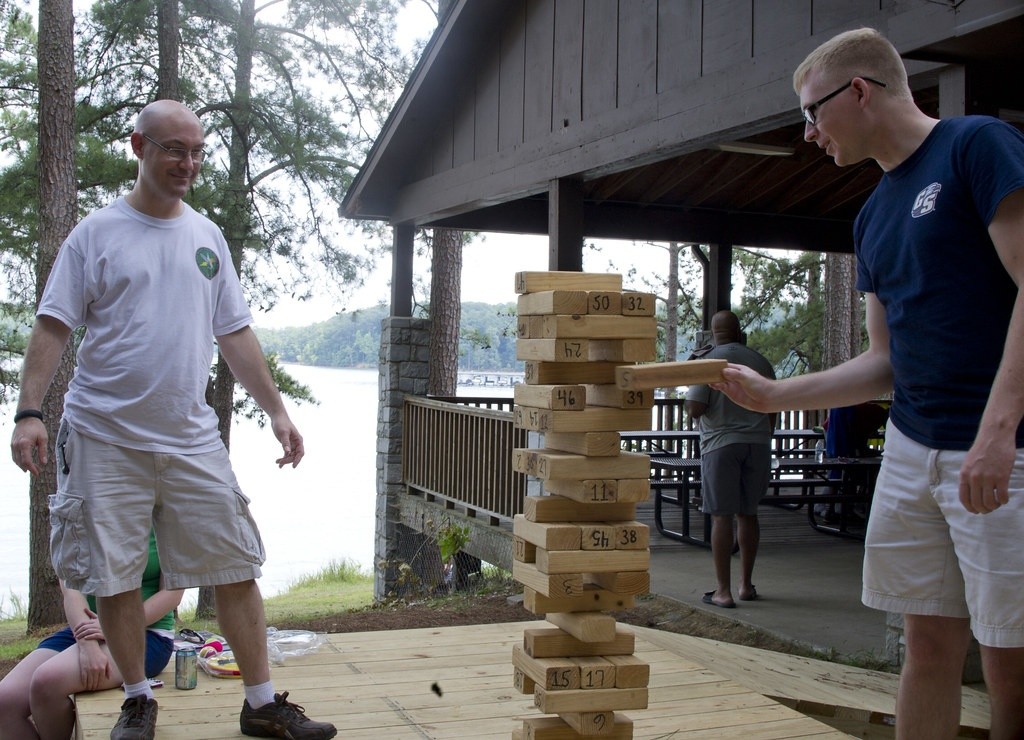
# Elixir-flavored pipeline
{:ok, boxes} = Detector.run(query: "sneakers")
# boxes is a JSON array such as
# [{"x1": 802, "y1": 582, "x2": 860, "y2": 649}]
[
  {"x1": 109, "y1": 693, "x2": 159, "y2": 740},
  {"x1": 239, "y1": 691, "x2": 337, "y2": 740}
]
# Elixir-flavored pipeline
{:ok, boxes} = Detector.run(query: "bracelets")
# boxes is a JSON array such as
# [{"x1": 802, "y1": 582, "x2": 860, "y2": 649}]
[{"x1": 14, "y1": 409, "x2": 44, "y2": 423}]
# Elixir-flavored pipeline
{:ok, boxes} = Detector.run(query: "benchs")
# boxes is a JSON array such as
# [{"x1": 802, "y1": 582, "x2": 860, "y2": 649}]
[
  {"x1": 771, "y1": 449, "x2": 826, "y2": 458},
  {"x1": 692, "y1": 493, "x2": 873, "y2": 554},
  {"x1": 650, "y1": 479, "x2": 842, "y2": 530}
]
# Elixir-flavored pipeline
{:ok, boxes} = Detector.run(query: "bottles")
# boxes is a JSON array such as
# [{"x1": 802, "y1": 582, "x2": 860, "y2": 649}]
[{"x1": 815, "y1": 440, "x2": 823, "y2": 463}]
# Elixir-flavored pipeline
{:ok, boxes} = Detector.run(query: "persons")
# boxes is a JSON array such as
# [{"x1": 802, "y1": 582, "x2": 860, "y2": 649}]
[
  {"x1": 684, "y1": 28, "x2": 1024, "y2": 740},
  {"x1": 0, "y1": 100, "x2": 337, "y2": 740}
]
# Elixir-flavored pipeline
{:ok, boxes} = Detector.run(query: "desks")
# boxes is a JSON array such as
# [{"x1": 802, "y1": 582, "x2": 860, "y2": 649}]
[
  {"x1": 618, "y1": 431, "x2": 825, "y2": 504},
  {"x1": 649, "y1": 456, "x2": 881, "y2": 554}
]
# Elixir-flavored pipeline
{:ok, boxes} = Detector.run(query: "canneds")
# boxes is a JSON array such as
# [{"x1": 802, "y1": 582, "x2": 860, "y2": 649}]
[{"x1": 175, "y1": 648, "x2": 197, "y2": 690}]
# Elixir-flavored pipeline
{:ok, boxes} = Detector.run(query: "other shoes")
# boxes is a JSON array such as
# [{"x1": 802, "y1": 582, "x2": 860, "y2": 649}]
[
  {"x1": 701, "y1": 589, "x2": 736, "y2": 609},
  {"x1": 738, "y1": 584, "x2": 757, "y2": 601}
]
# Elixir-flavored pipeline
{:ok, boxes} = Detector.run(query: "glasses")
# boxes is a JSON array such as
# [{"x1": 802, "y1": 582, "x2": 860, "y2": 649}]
[
  {"x1": 801, "y1": 77, "x2": 886, "y2": 126},
  {"x1": 143, "y1": 134, "x2": 215, "y2": 159}
]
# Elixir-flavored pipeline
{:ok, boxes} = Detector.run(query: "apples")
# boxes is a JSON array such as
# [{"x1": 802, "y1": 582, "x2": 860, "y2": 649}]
[{"x1": 200, "y1": 638, "x2": 219, "y2": 657}]
